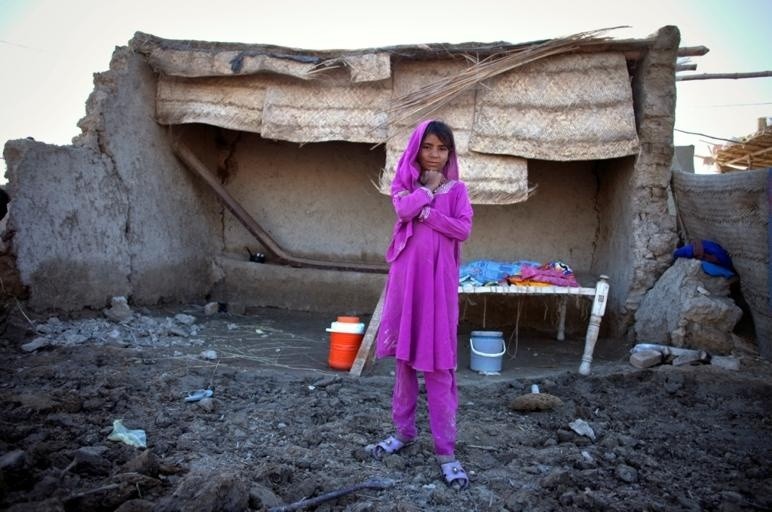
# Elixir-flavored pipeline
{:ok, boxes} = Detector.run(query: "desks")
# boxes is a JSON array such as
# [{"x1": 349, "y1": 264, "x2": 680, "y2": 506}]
[{"x1": 456, "y1": 273, "x2": 610, "y2": 374}]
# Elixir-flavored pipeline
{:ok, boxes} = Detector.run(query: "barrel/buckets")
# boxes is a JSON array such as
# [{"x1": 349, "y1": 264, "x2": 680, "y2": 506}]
[
  {"x1": 325, "y1": 314, "x2": 365, "y2": 369},
  {"x1": 469, "y1": 331, "x2": 507, "y2": 373}
]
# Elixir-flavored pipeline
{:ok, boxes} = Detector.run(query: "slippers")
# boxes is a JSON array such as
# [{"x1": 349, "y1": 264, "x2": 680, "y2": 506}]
[
  {"x1": 373, "y1": 434, "x2": 415, "y2": 462},
  {"x1": 438, "y1": 459, "x2": 469, "y2": 492}
]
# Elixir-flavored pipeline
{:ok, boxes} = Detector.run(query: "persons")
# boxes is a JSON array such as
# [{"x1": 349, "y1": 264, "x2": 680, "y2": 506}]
[{"x1": 370, "y1": 120, "x2": 474, "y2": 494}]
